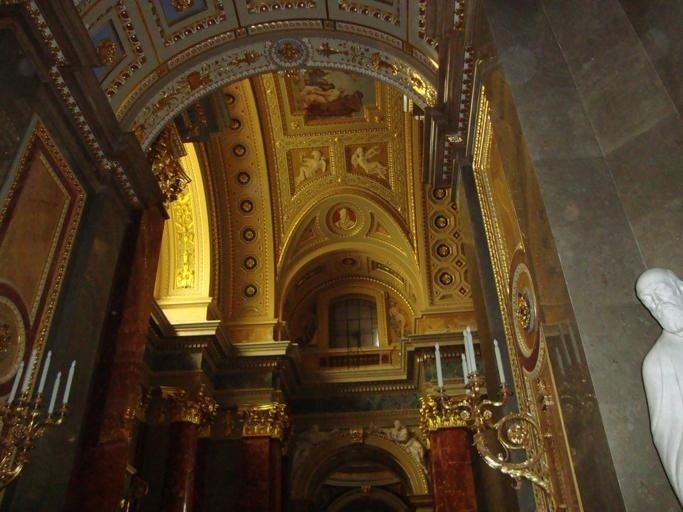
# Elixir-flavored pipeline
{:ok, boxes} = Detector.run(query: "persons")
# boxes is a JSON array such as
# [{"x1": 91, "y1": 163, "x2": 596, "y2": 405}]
[
  {"x1": 635, "y1": 266, "x2": 682, "y2": 509},
  {"x1": 388, "y1": 301, "x2": 406, "y2": 340},
  {"x1": 287, "y1": 422, "x2": 338, "y2": 482},
  {"x1": 294, "y1": 149, "x2": 328, "y2": 188},
  {"x1": 292, "y1": 303, "x2": 316, "y2": 349},
  {"x1": 377, "y1": 418, "x2": 429, "y2": 477},
  {"x1": 299, "y1": 67, "x2": 364, "y2": 121},
  {"x1": 334, "y1": 208, "x2": 356, "y2": 230},
  {"x1": 350, "y1": 145, "x2": 387, "y2": 180}
]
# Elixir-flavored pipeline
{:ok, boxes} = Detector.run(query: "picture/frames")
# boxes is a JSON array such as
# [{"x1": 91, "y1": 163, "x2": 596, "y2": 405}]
[
  {"x1": 0, "y1": 109, "x2": 89, "y2": 411},
  {"x1": 471, "y1": 84, "x2": 585, "y2": 512}
]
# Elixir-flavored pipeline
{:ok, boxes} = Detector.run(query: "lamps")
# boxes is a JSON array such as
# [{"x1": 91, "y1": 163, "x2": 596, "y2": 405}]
[
  {"x1": 0, "y1": 347, "x2": 77, "y2": 491},
  {"x1": 435, "y1": 325, "x2": 552, "y2": 498}
]
